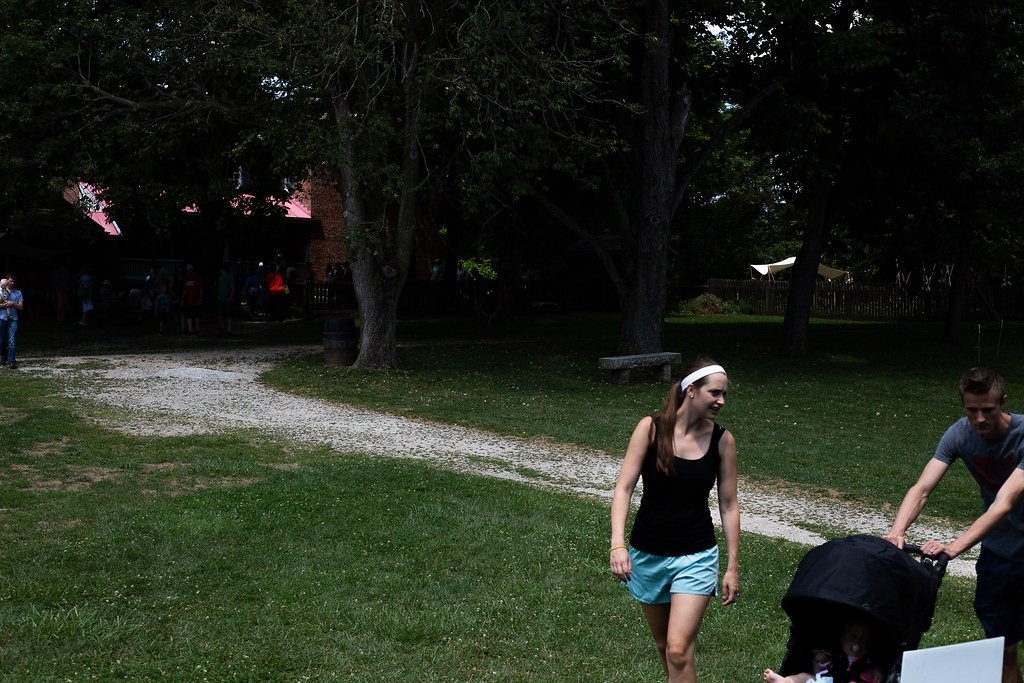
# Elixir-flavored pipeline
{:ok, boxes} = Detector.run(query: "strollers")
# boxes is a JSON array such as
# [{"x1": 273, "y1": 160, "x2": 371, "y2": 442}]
[{"x1": 777, "y1": 531, "x2": 947, "y2": 682}]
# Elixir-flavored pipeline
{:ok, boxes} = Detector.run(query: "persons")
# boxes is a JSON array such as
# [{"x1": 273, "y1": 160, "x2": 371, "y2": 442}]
[
  {"x1": 0, "y1": 278, "x2": 14, "y2": 321},
  {"x1": 764, "y1": 620, "x2": 883, "y2": 683},
  {"x1": 22, "y1": 257, "x2": 293, "y2": 336},
  {"x1": 0, "y1": 273, "x2": 23, "y2": 369},
  {"x1": 609, "y1": 359, "x2": 742, "y2": 681},
  {"x1": 881, "y1": 365, "x2": 1024, "y2": 682}
]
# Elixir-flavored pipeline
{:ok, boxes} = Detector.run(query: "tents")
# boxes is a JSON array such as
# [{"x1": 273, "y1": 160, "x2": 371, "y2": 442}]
[{"x1": 750, "y1": 256, "x2": 850, "y2": 314}]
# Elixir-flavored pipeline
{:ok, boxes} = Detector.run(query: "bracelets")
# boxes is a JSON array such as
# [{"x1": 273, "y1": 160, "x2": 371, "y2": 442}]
[{"x1": 609, "y1": 546, "x2": 629, "y2": 552}]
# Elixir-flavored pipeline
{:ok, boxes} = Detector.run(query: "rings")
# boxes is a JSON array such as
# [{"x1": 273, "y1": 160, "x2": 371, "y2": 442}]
[{"x1": 929, "y1": 549, "x2": 933, "y2": 553}]
